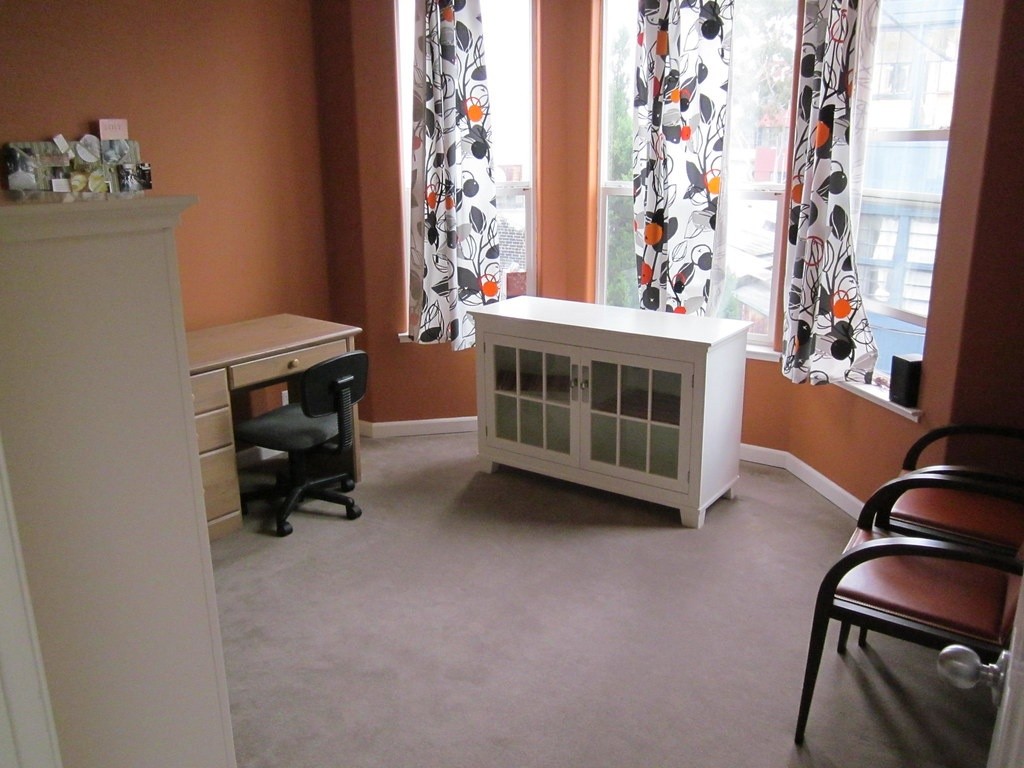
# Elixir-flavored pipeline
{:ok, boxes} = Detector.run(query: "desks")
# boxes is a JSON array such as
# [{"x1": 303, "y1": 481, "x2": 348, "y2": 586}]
[{"x1": 184, "y1": 311, "x2": 364, "y2": 539}]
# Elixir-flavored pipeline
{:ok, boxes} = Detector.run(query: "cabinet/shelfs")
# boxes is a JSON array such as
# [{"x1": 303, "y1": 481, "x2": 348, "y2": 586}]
[
  {"x1": 467, "y1": 296, "x2": 751, "y2": 529},
  {"x1": 0, "y1": 195, "x2": 236, "y2": 767}
]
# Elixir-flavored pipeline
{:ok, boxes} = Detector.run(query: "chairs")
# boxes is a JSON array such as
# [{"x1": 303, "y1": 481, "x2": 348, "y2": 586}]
[
  {"x1": 793, "y1": 419, "x2": 1023, "y2": 747},
  {"x1": 235, "y1": 351, "x2": 370, "y2": 536}
]
